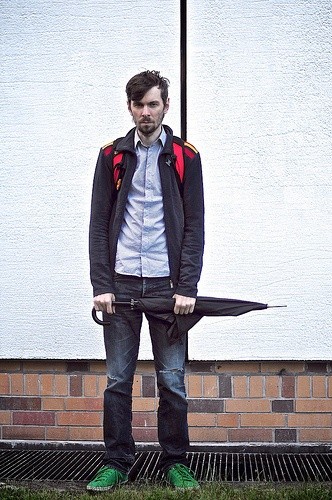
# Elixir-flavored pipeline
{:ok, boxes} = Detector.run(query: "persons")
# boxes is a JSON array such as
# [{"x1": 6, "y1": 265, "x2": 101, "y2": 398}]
[{"x1": 87, "y1": 70, "x2": 205, "y2": 491}]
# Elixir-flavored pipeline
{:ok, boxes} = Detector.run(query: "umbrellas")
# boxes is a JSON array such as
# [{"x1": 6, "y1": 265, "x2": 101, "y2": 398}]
[{"x1": 91, "y1": 296, "x2": 287, "y2": 347}]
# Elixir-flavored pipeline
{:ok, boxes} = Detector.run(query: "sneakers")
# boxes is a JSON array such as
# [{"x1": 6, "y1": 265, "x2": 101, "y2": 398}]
[
  {"x1": 168, "y1": 463, "x2": 201, "y2": 492},
  {"x1": 86, "y1": 464, "x2": 129, "y2": 492}
]
